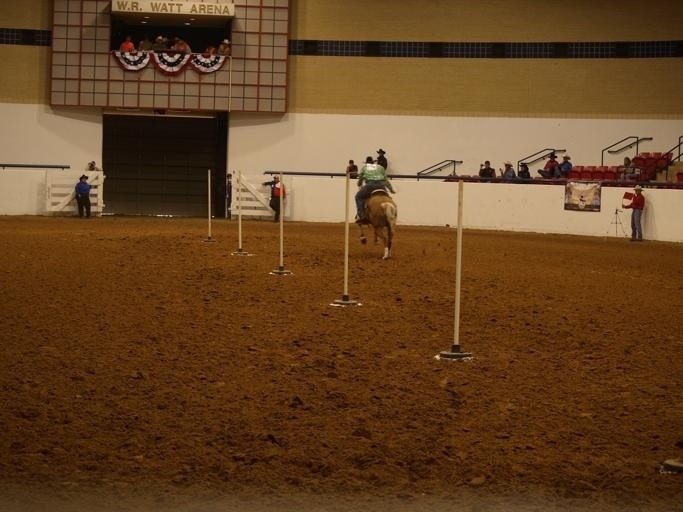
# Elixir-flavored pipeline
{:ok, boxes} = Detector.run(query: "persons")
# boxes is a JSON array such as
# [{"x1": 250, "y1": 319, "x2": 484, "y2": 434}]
[
  {"x1": 88, "y1": 161, "x2": 100, "y2": 206},
  {"x1": 225, "y1": 173, "x2": 232, "y2": 219},
  {"x1": 349, "y1": 160, "x2": 358, "y2": 179},
  {"x1": 620, "y1": 157, "x2": 642, "y2": 179},
  {"x1": 354, "y1": 156, "x2": 396, "y2": 223},
  {"x1": 262, "y1": 175, "x2": 286, "y2": 222},
  {"x1": 622, "y1": 185, "x2": 645, "y2": 241},
  {"x1": 120, "y1": 35, "x2": 231, "y2": 55},
  {"x1": 376, "y1": 149, "x2": 387, "y2": 169},
  {"x1": 75, "y1": 175, "x2": 93, "y2": 219},
  {"x1": 479, "y1": 153, "x2": 572, "y2": 184}
]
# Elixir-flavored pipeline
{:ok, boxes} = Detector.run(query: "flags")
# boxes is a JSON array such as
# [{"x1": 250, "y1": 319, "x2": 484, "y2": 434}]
[{"x1": 623, "y1": 192, "x2": 633, "y2": 200}]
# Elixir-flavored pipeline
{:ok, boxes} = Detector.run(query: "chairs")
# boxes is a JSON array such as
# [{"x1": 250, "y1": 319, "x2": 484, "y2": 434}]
[{"x1": 568, "y1": 151, "x2": 683, "y2": 190}]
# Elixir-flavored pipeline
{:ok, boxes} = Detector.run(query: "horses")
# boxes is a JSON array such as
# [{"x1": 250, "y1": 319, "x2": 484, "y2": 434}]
[{"x1": 357, "y1": 185, "x2": 398, "y2": 260}]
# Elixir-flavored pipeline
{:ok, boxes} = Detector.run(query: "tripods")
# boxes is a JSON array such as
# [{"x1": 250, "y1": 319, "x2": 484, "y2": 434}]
[{"x1": 605, "y1": 209, "x2": 628, "y2": 238}]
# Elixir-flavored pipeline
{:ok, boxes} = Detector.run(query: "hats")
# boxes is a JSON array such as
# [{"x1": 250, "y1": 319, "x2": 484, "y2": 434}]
[
  {"x1": 79, "y1": 175, "x2": 88, "y2": 181},
  {"x1": 632, "y1": 185, "x2": 644, "y2": 192},
  {"x1": 349, "y1": 148, "x2": 385, "y2": 166},
  {"x1": 504, "y1": 153, "x2": 572, "y2": 169}
]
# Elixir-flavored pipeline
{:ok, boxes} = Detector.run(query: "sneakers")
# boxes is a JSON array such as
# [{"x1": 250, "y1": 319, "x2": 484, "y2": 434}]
[{"x1": 355, "y1": 217, "x2": 367, "y2": 224}]
